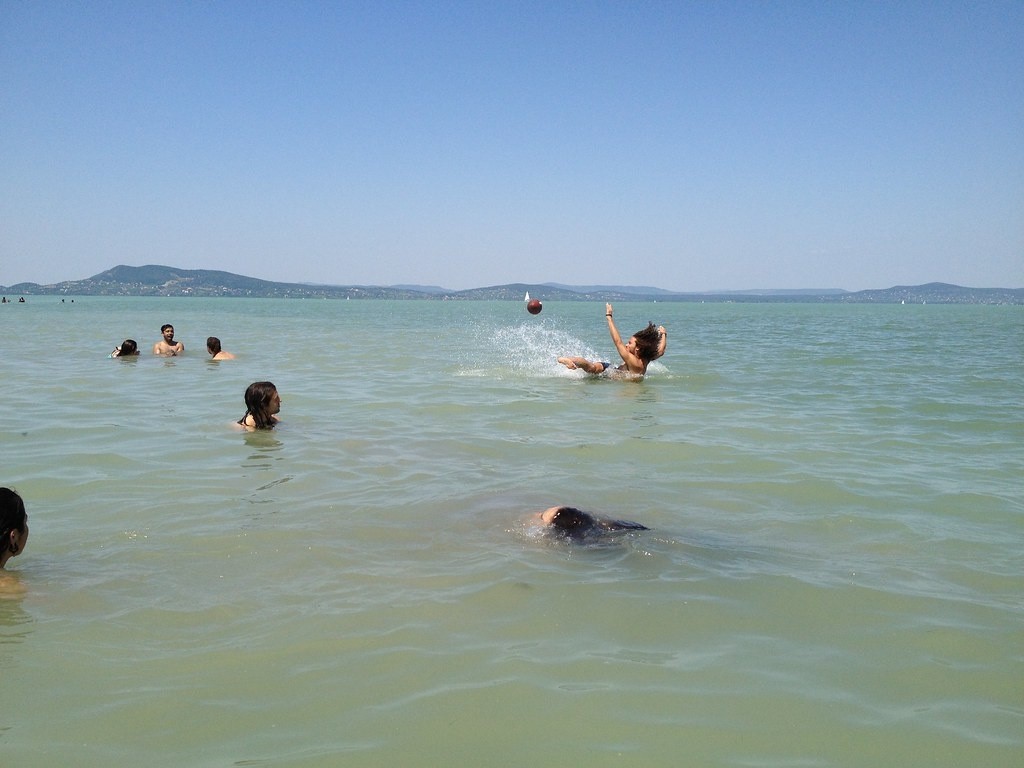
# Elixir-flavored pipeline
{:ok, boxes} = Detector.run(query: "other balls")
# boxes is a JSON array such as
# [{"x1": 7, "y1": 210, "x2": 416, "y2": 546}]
[{"x1": 527, "y1": 300, "x2": 542, "y2": 315}]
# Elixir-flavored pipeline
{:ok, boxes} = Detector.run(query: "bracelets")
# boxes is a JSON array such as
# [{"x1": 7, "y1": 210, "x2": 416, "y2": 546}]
[{"x1": 605, "y1": 314, "x2": 612, "y2": 317}]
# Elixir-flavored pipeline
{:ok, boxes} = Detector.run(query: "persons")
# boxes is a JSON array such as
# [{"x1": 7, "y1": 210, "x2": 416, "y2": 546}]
[
  {"x1": 0, "y1": 487, "x2": 29, "y2": 569},
  {"x1": 558, "y1": 301, "x2": 667, "y2": 375},
  {"x1": 108, "y1": 339, "x2": 141, "y2": 357},
  {"x1": 237, "y1": 381, "x2": 283, "y2": 427},
  {"x1": 154, "y1": 324, "x2": 184, "y2": 354},
  {"x1": 2, "y1": 296, "x2": 74, "y2": 303},
  {"x1": 543, "y1": 506, "x2": 593, "y2": 529},
  {"x1": 207, "y1": 337, "x2": 233, "y2": 359}
]
